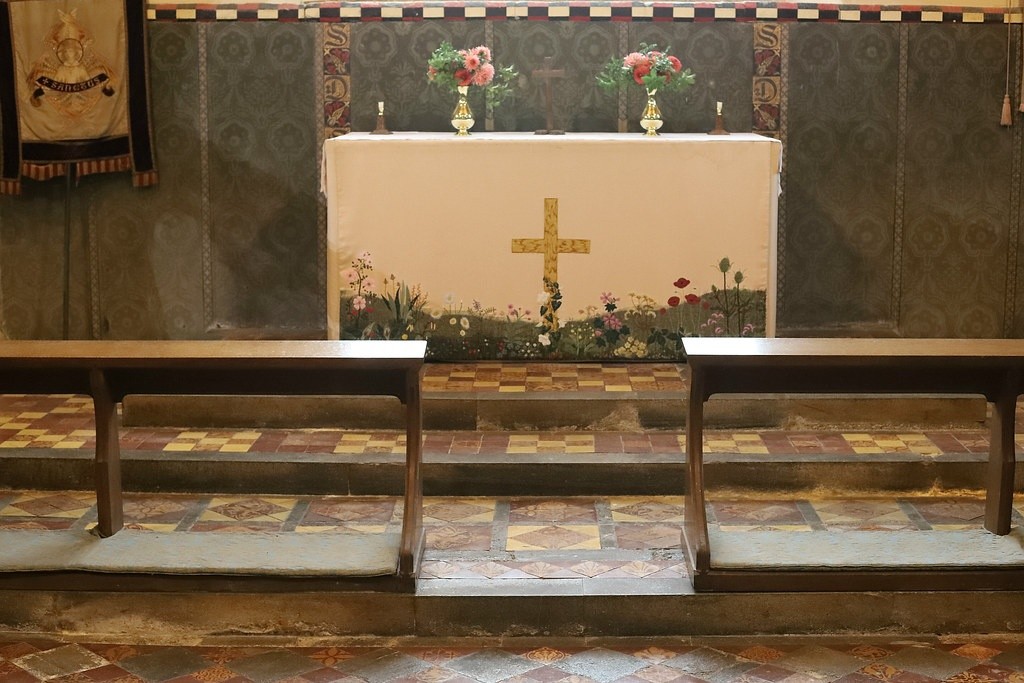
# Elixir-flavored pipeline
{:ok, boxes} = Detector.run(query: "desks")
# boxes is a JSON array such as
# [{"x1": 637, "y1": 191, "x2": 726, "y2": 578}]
[
  {"x1": 321, "y1": 118, "x2": 783, "y2": 366},
  {"x1": 680, "y1": 336, "x2": 1024, "y2": 595},
  {"x1": 0, "y1": 338, "x2": 429, "y2": 593}
]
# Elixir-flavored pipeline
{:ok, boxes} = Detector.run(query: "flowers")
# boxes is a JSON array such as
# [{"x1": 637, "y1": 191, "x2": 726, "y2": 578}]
[
  {"x1": 425, "y1": 40, "x2": 519, "y2": 107},
  {"x1": 593, "y1": 42, "x2": 696, "y2": 93}
]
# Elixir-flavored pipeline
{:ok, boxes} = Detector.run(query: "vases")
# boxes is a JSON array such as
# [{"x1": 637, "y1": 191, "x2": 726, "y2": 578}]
[
  {"x1": 450, "y1": 84, "x2": 476, "y2": 136},
  {"x1": 639, "y1": 87, "x2": 664, "y2": 137}
]
